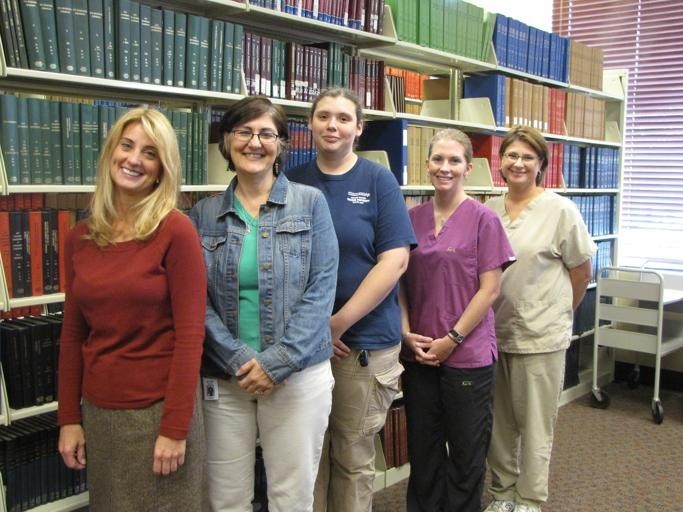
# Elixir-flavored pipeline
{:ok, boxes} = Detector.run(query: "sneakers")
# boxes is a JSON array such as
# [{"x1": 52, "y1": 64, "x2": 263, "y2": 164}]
[
  {"x1": 482, "y1": 500, "x2": 515, "y2": 512},
  {"x1": 513, "y1": 504, "x2": 541, "y2": 512}
]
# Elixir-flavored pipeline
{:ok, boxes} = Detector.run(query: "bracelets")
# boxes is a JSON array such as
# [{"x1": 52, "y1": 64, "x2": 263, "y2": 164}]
[{"x1": 404, "y1": 331, "x2": 410, "y2": 343}]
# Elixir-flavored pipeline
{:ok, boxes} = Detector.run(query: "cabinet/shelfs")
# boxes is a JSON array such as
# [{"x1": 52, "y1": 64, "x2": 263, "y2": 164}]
[{"x1": 0, "y1": 0, "x2": 632, "y2": 512}]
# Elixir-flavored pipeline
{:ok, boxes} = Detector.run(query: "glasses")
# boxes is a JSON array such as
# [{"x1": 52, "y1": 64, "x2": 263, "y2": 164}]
[
  {"x1": 503, "y1": 152, "x2": 534, "y2": 161},
  {"x1": 234, "y1": 129, "x2": 278, "y2": 145}
]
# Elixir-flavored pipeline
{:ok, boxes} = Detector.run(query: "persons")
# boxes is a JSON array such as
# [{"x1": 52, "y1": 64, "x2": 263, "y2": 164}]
[
  {"x1": 187, "y1": 96, "x2": 340, "y2": 511},
  {"x1": 56, "y1": 109, "x2": 206, "y2": 511},
  {"x1": 399, "y1": 128, "x2": 517, "y2": 511},
  {"x1": 483, "y1": 126, "x2": 601, "y2": 511},
  {"x1": 285, "y1": 88, "x2": 416, "y2": 511}
]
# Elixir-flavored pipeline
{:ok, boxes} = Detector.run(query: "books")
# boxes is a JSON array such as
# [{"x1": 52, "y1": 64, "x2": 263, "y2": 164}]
[
  {"x1": 0, "y1": 285, "x2": 89, "y2": 511},
  {"x1": 383, "y1": 406, "x2": 408, "y2": 469},
  {"x1": 0, "y1": 0, "x2": 620, "y2": 298}
]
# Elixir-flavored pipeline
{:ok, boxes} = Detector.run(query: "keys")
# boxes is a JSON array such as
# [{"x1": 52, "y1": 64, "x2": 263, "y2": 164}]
[{"x1": 357, "y1": 346, "x2": 368, "y2": 366}]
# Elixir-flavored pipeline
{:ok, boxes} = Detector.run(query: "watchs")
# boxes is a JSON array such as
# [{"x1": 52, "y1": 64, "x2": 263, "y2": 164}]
[{"x1": 447, "y1": 327, "x2": 464, "y2": 343}]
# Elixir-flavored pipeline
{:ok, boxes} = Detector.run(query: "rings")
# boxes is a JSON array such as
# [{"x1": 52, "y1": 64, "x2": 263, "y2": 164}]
[{"x1": 255, "y1": 389, "x2": 263, "y2": 395}]
